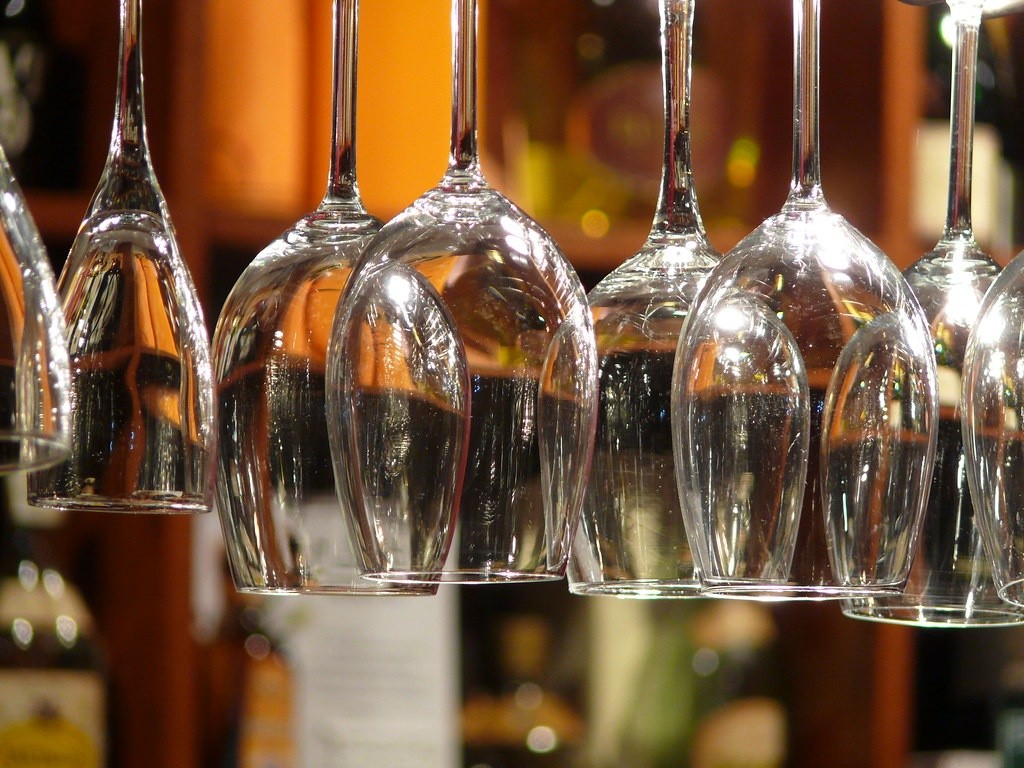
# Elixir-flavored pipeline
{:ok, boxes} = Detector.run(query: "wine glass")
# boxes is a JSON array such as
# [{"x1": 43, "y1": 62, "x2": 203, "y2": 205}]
[
  {"x1": 26, "y1": 1, "x2": 218, "y2": 514},
  {"x1": 210, "y1": 0, "x2": 473, "y2": 597},
  {"x1": 535, "y1": 0, "x2": 812, "y2": 601},
  {"x1": 325, "y1": 1, "x2": 599, "y2": 587},
  {"x1": 820, "y1": 0, "x2": 1024, "y2": 629},
  {"x1": 672, "y1": 0, "x2": 939, "y2": 605}
]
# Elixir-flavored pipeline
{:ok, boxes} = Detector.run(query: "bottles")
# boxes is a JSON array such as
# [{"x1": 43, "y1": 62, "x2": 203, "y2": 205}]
[
  {"x1": 198, "y1": 544, "x2": 294, "y2": 768},
  {"x1": 0, "y1": 469, "x2": 105, "y2": 767}
]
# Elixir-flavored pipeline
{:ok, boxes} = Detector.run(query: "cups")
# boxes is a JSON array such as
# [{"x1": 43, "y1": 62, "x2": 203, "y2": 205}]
[
  {"x1": 0, "y1": 142, "x2": 74, "y2": 475},
  {"x1": 959, "y1": 250, "x2": 1024, "y2": 608}
]
